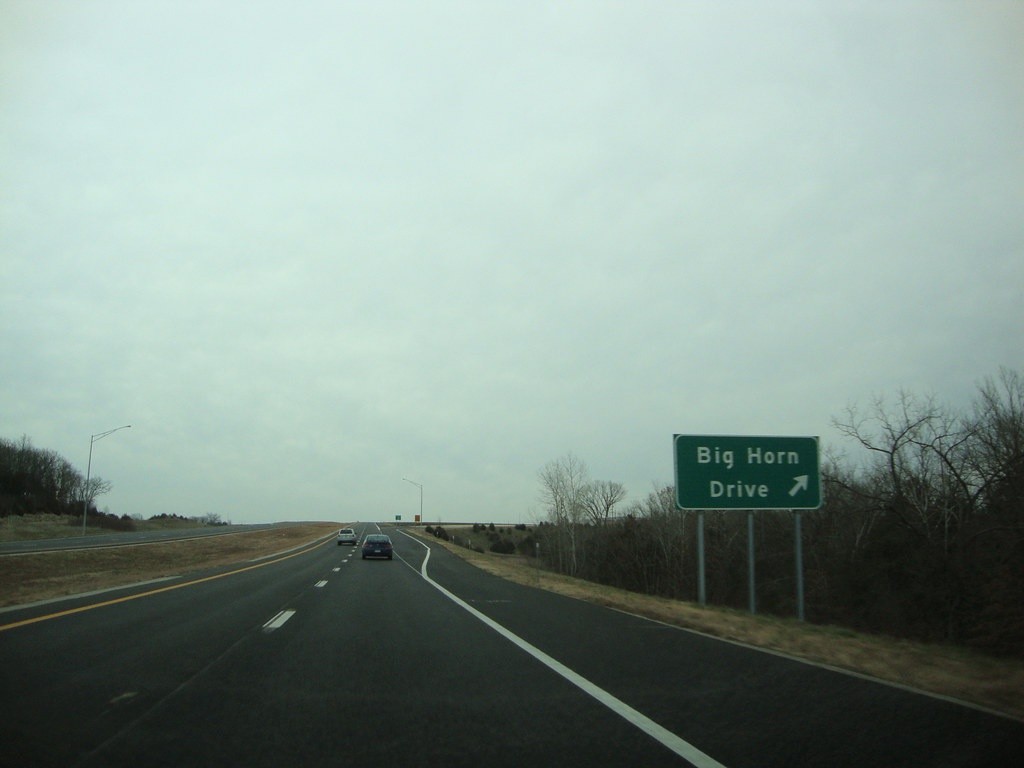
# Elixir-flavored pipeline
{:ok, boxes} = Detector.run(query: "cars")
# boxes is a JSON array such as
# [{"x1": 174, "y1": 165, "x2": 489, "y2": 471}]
[
  {"x1": 361, "y1": 533, "x2": 394, "y2": 560},
  {"x1": 337, "y1": 529, "x2": 358, "y2": 545}
]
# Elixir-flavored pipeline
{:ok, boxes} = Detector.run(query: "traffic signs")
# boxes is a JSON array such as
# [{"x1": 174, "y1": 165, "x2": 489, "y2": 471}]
[{"x1": 671, "y1": 432, "x2": 825, "y2": 511}]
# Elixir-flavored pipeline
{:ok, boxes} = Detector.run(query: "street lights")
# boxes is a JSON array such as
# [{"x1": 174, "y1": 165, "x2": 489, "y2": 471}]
[
  {"x1": 81, "y1": 424, "x2": 132, "y2": 536},
  {"x1": 402, "y1": 478, "x2": 423, "y2": 529}
]
